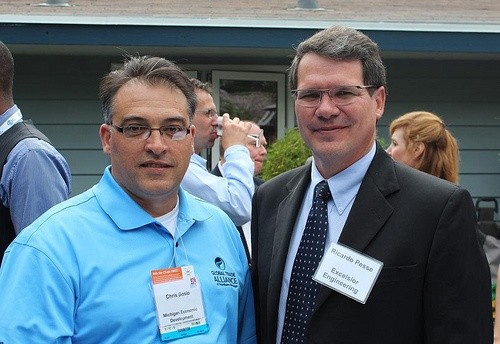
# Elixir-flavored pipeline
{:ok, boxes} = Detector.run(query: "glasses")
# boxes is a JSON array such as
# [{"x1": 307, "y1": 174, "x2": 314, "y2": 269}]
[
  {"x1": 111, "y1": 124, "x2": 190, "y2": 141},
  {"x1": 191, "y1": 109, "x2": 219, "y2": 119},
  {"x1": 290, "y1": 84, "x2": 377, "y2": 108}
]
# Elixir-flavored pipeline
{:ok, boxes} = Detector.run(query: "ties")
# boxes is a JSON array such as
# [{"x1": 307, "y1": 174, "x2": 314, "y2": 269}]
[{"x1": 281, "y1": 180, "x2": 334, "y2": 344}]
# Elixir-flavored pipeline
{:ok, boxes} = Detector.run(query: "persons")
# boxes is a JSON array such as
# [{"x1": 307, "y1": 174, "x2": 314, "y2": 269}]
[
  {"x1": 0, "y1": 45, "x2": 256, "y2": 344},
  {"x1": 210, "y1": 119, "x2": 268, "y2": 268},
  {"x1": 180, "y1": 78, "x2": 256, "y2": 266},
  {"x1": 386, "y1": 110, "x2": 459, "y2": 183},
  {"x1": 0, "y1": 41, "x2": 70, "y2": 267},
  {"x1": 250, "y1": 25, "x2": 494, "y2": 344}
]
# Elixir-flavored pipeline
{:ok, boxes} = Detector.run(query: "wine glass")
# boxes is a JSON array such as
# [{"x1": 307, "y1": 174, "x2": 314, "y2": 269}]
[{"x1": 217, "y1": 116, "x2": 263, "y2": 148}]
